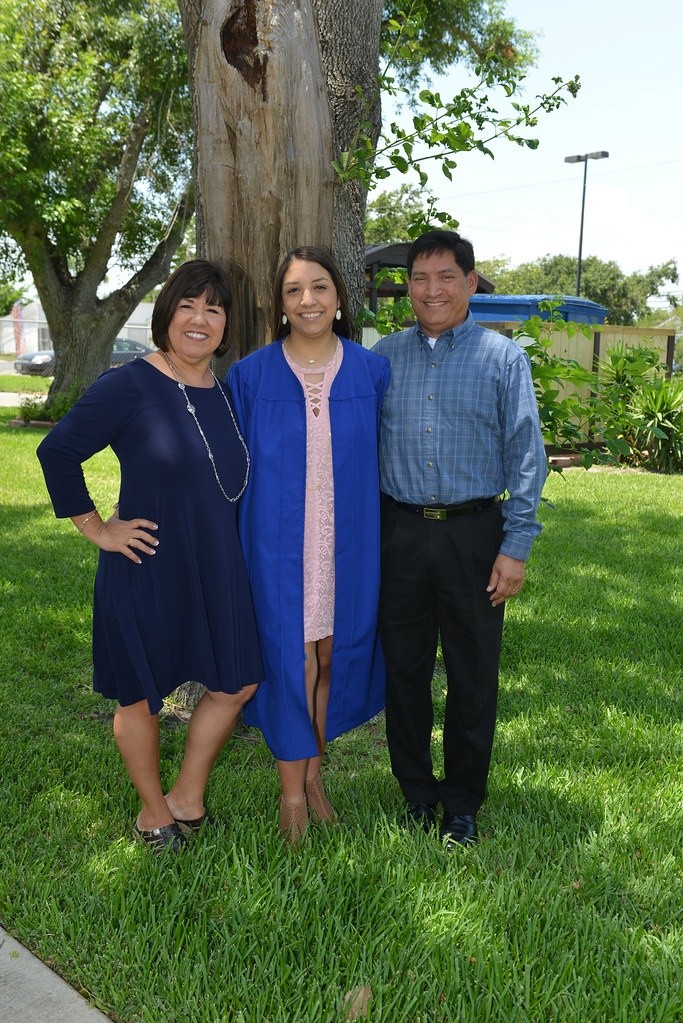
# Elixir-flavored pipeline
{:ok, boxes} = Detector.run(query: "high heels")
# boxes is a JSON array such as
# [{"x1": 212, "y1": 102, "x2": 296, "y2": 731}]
[
  {"x1": 279, "y1": 792, "x2": 309, "y2": 858},
  {"x1": 305, "y1": 773, "x2": 340, "y2": 828}
]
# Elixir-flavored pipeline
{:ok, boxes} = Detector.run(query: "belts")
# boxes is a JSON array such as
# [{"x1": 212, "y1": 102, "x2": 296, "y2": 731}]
[{"x1": 383, "y1": 496, "x2": 501, "y2": 521}]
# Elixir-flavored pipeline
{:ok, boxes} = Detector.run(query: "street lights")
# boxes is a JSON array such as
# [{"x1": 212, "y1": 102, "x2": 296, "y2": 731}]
[{"x1": 564, "y1": 151, "x2": 609, "y2": 297}]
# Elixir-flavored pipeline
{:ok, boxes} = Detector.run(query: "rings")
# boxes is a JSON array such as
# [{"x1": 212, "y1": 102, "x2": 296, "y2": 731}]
[
  {"x1": 512, "y1": 589, "x2": 517, "y2": 592},
  {"x1": 128, "y1": 537, "x2": 133, "y2": 545}
]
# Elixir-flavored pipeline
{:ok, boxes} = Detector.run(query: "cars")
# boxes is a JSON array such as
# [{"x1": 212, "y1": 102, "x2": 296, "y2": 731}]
[{"x1": 15, "y1": 337, "x2": 156, "y2": 377}]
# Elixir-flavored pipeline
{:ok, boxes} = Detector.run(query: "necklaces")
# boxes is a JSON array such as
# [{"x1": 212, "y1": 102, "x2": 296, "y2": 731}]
[
  {"x1": 284, "y1": 331, "x2": 338, "y2": 363},
  {"x1": 158, "y1": 349, "x2": 250, "y2": 502}
]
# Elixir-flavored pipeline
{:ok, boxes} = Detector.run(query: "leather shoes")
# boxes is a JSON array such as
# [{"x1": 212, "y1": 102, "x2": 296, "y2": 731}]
[
  {"x1": 439, "y1": 810, "x2": 479, "y2": 853},
  {"x1": 399, "y1": 801, "x2": 437, "y2": 841}
]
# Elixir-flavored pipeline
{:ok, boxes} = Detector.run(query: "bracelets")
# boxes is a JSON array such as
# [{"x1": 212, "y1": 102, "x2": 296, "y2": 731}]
[{"x1": 80, "y1": 511, "x2": 97, "y2": 533}]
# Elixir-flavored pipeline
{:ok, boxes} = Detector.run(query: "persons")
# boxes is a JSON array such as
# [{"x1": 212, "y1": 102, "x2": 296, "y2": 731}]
[
  {"x1": 224, "y1": 245, "x2": 385, "y2": 847},
  {"x1": 368, "y1": 231, "x2": 551, "y2": 847},
  {"x1": 36, "y1": 254, "x2": 265, "y2": 856}
]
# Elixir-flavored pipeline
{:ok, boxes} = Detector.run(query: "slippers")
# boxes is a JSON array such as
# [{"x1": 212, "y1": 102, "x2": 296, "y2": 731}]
[
  {"x1": 133, "y1": 817, "x2": 192, "y2": 860},
  {"x1": 172, "y1": 807, "x2": 230, "y2": 838}
]
